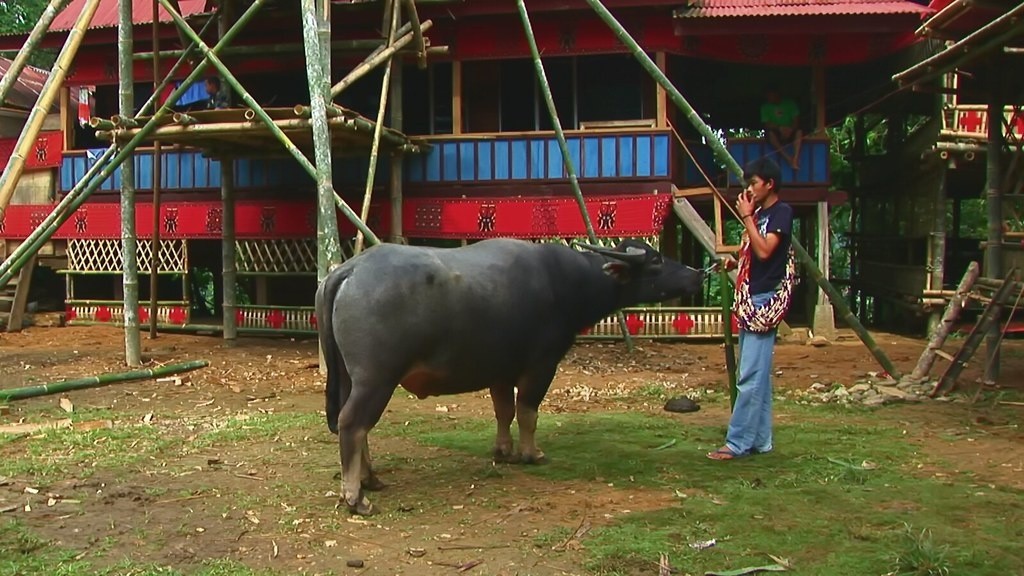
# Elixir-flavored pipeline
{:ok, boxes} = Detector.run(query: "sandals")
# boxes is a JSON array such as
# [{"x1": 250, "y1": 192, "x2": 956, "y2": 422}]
[{"x1": 705, "y1": 447, "x2": 738, "y2": 460}]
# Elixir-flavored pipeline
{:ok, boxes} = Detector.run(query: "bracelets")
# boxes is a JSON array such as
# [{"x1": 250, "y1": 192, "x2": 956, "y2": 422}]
[{"x1": 742, "y1": 212, "x2": 755, "y2": 219}]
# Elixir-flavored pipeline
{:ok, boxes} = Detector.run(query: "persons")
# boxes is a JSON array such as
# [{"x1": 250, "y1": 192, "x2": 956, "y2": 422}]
[
  {"x1": 705, "y1": 157, "x2": 794, "y2": 460},
  {"x1": 203, "y1": 80, "x2": 225, "y2": 110}
]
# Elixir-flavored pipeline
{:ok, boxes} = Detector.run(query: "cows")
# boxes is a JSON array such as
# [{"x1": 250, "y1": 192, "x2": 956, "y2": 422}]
[{"x1": 315, "y1": 238, "x2": 706, "y2": 517}]
